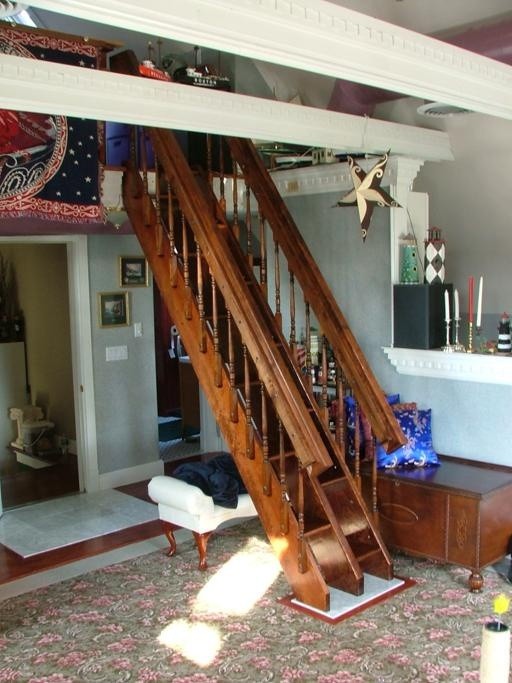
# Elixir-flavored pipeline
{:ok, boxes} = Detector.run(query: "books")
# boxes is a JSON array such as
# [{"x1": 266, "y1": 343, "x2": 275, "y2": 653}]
[{"x1": 275, "y1": 155, "x2": 312, "y2": 164}]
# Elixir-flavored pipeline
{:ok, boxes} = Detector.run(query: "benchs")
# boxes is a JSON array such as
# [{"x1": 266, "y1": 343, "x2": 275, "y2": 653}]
[{"x1": 147, "y1": 474, "x2": 260, "y2": 572}]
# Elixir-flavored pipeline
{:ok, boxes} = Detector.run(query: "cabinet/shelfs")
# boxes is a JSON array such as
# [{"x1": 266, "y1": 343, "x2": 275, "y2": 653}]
[
  {"x1": 178, "y1": 355, "x2": 200, "y2": 440},
  {"x1": 309, "y1": 441, "x2": 511, "y2": 594}
]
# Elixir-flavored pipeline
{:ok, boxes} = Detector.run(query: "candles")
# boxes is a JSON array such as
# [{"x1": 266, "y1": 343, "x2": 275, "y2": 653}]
[{"x1": 444, "y1": 275, "x2": 484, "y2": 327}]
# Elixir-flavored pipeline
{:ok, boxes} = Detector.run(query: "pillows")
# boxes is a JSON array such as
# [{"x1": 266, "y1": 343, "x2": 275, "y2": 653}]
[{"x1": 330, "y1": 391, "x2": 443, "y2": 472}]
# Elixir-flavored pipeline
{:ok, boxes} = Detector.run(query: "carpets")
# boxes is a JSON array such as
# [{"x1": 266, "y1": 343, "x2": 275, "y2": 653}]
[{"x1": 0, "y1": 487, "x2": 512, "y2": 683}]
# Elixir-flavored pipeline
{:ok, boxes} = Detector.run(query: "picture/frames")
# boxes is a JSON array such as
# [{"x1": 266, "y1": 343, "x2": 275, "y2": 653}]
[{"x1": 96, "y1": 253, "x2": 150, "y2": 330}]
